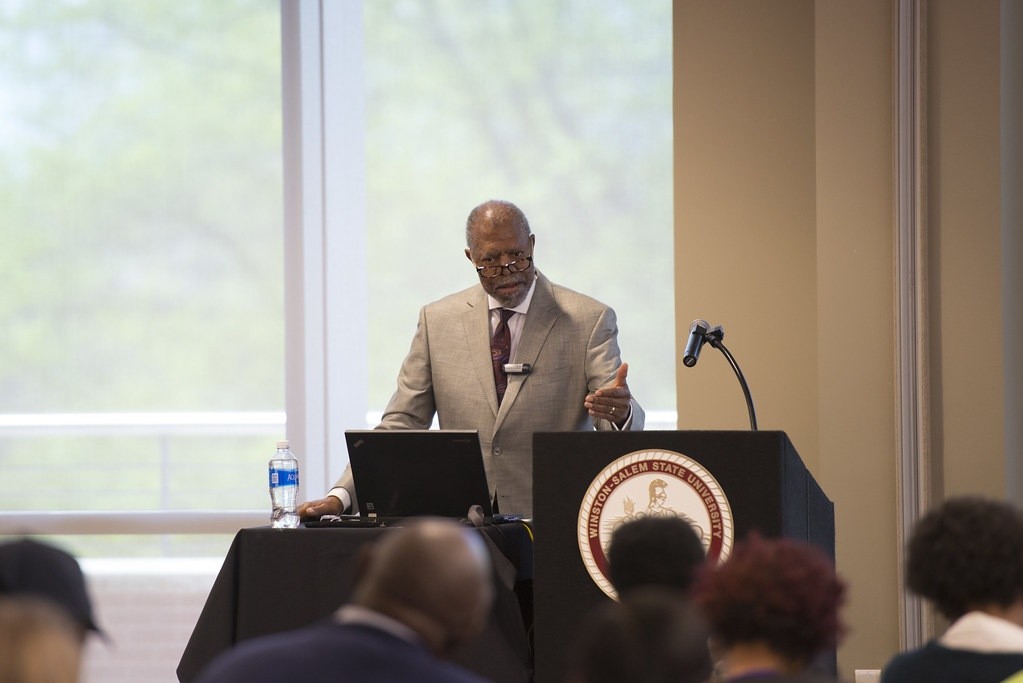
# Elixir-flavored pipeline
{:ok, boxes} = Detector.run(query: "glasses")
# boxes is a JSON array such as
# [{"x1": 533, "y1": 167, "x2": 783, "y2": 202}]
[{"x1": 470, "y1": 240, "x2": 533, "y2": 278}]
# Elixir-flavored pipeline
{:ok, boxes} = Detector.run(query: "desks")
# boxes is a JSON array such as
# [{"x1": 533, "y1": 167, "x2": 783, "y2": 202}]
[{"x1": 175, "y1": 523, "x2": 533, "y2": 683}]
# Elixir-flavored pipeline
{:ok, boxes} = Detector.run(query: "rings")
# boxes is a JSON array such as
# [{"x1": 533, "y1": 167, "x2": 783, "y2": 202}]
[{"x1": 609, "y1": 407, "x2": 615, "y2": 414}]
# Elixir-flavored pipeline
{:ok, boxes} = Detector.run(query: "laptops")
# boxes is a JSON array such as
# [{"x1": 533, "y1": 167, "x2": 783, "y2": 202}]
[{"x1": 344, "y1": 429, "x2": 524, "y2": 525}]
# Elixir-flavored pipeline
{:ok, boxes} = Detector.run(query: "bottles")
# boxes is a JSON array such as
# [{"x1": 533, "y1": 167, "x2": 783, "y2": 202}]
[{"x1": 269, "y1": 441, "x2": 300, "y2": 529}]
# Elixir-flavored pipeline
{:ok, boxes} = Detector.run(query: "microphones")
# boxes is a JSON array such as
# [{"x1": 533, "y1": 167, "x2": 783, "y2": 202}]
[{"x1": 682, "y1": 319, "x2": 711, "y2": 367}]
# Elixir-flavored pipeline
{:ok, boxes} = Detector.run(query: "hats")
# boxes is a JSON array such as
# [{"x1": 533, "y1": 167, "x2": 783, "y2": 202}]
[{"x1": 0, "y1": 539, "x2": 102, "y2": 635}]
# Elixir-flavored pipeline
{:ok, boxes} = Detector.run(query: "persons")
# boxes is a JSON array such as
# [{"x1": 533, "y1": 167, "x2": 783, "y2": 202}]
[
  {"x1": 0, "y1": 538, "x2": 112, "y2": 683},
  {"x1": 296, "y1": 200, "x2": 645, "y2": 518},
  {"x1": 877, "y1": 496, "x2": 1023, "y2": 683},
  {"x1": 190, "y1": 518, "x2": 493, "y2": 683},
  {"x1": 548, "y1": 516, "x2": 847, "y2": 683}
]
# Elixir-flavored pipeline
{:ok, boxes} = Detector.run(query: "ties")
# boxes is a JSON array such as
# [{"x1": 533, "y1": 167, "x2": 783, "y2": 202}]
[{"x1": 490, "y1": 307, "x2": 515, "y2": 407}]
